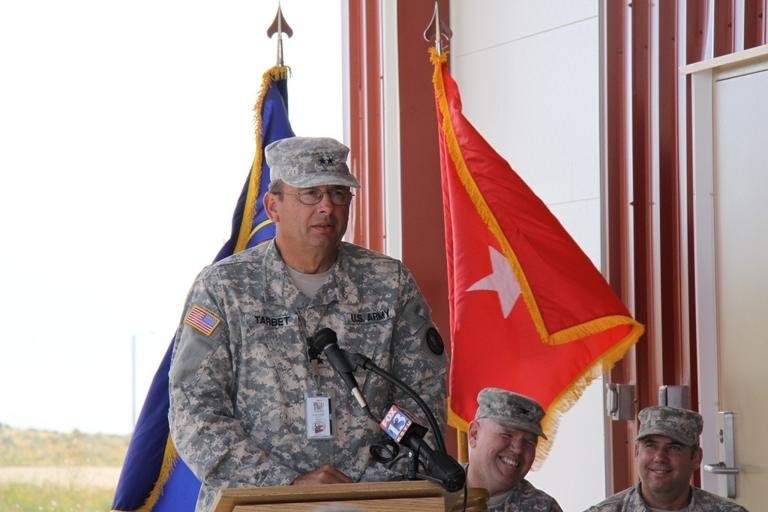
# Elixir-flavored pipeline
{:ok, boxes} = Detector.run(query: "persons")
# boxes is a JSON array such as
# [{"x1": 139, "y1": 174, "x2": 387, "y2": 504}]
[
  {"x1": 167, "y1": 137, "x2": 450, "y2": 512},
  {"x1": 454, "y1": 386, "x2": 564, "y2": 512},
  {"x1": 584, "y1": 406, "x2": 747, "y2": 512}
]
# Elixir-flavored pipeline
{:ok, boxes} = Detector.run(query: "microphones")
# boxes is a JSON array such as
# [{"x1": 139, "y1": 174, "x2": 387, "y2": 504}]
[
  {"x1": 378, "y1": 401, "x2": 468, "y2": 491},
  {"x1": 313, "y1": 328, "x2": 372, "y2": 420}
]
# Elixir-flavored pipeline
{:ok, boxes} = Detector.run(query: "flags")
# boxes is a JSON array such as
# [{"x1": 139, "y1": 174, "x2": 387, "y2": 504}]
[
  {"x1": 108, "y1": 66, "x2": 297, "y2": 512},
  {"x1": 428, "y1": 44, "x2": 647, "y2": 475}
]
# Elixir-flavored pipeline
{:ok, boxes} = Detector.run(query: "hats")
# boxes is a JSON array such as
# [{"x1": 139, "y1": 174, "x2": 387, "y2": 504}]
[
  {"x1": 634, "y1": 405, "x2": 704, "y2": 447},
  {"x1": 264, "y1": 137, "x2": 363, "y2": 189},
  {"x1": 277, "y1": 190, "x2": 355, "y2": 207},
  {"x1": 473, "y1": 387, "x2": 548, "y2": 441}
]
